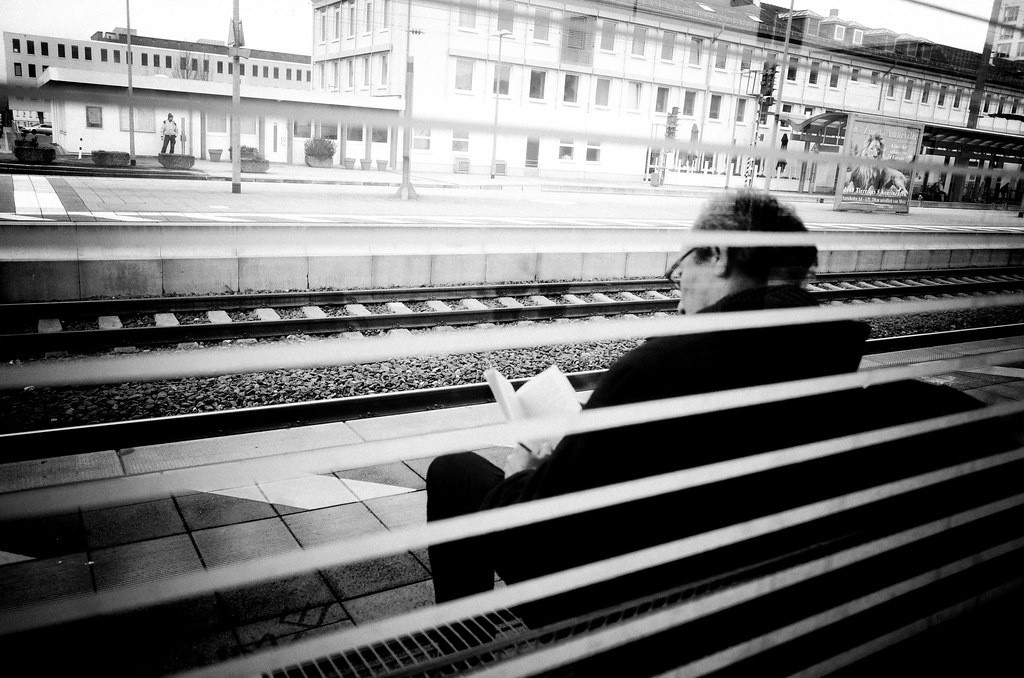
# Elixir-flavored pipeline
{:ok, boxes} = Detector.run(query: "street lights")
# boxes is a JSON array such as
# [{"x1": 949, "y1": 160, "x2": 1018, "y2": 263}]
[{"x1": 489, "y1": 29, "x2": 513, "y2": 179}]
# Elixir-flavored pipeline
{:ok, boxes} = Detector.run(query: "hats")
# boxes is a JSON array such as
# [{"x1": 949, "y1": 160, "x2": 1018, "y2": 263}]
[{"x1": 167, "y1": 113, "x2": 173, "y2": 120}]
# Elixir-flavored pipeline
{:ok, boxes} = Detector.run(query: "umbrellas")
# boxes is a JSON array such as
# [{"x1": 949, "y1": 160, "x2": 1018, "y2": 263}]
[
  {"x1": 776, "y1": 133, "x2": 789, "y2": 175},
  {"x1": 688, "y1": 123, "x2": 698, "y2": 167}
]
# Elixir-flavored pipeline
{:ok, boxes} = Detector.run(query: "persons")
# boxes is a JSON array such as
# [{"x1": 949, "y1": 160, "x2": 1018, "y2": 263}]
[
  {"x1": 931, "y1": 180, "x2": 943, "y2": 194},
  {"x1": 160, "y1": 113, "x2": 178, "y2": 154},
  {"x1": 1001, "y1": 182, "x2": 1010, "y2": 199},
  {"x1": 979, "y1": 182, "x2": 987, "y2": 200},
  {"x1": 427, "y1": 193, "x2": 871, "y2": 673}
]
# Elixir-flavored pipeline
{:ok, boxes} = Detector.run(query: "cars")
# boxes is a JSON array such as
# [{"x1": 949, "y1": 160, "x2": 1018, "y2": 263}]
[{"x1": 21, "y1": 123, "x2": 54, "y2": 137}]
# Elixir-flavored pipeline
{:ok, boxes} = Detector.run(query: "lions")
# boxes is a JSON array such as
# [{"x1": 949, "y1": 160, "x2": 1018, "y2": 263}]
[{"x1": 843, "y1": 133, "x2": 909, "y2": 199}]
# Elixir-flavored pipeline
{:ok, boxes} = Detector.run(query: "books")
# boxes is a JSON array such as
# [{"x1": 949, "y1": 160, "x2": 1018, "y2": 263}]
[{"x1": 483, "y1": 365, "x2": 583, "y2": 455}]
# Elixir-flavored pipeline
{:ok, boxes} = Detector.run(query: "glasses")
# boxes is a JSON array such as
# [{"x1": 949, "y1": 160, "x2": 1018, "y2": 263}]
[{"x1": 665, "y1": 249, "x2": 702, "y2": 287}]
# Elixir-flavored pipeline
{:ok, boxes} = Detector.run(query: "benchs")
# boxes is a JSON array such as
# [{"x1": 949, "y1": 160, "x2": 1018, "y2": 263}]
[{"x1": 260, "y1": 507, "x2": 907, "y2": 678}]
